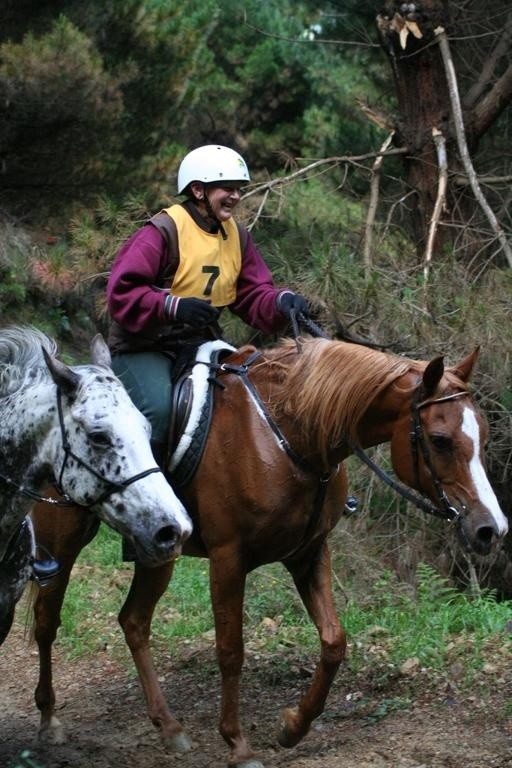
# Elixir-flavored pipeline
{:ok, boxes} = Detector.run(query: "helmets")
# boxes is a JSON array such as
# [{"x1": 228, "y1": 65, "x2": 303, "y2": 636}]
[{"x1": 177, "y1": 144, "x2": 250, "y2": 198}]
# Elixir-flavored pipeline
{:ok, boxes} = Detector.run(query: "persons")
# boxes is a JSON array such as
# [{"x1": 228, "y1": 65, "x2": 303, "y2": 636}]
[{"x1": 106, "y1": 144, "x2": 358, "y2": 561}]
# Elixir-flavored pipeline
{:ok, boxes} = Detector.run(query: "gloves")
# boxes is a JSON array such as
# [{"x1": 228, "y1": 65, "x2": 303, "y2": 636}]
[
  {"x1": 177, "y1": 297, "x2": 218, "y2": 328},
  {"x1": 280, "y1": 293, "x2": 307, "y2": 318}
]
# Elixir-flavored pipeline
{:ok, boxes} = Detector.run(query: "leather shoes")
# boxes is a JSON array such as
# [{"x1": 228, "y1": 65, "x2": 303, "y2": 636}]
[{"x1": 30, "y1": 557, "x2": 62, "y2": 581}]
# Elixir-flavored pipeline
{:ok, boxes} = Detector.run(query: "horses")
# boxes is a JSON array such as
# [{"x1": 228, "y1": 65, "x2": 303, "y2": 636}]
[
  {"x1": 0, "y1": 319, "x2": 197, "y2": 646},
  {"x1": 20, "y1": 332, "x2": 511, "y2": 768}
]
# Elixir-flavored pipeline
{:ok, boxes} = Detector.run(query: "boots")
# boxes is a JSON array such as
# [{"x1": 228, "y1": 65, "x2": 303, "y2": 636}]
[
  {"x1": 122, "y1": 437, "x2": 162, "y2": 561},
  {"x1": 344, "y1": 496, "x2": 357, "y2": 515}
]
